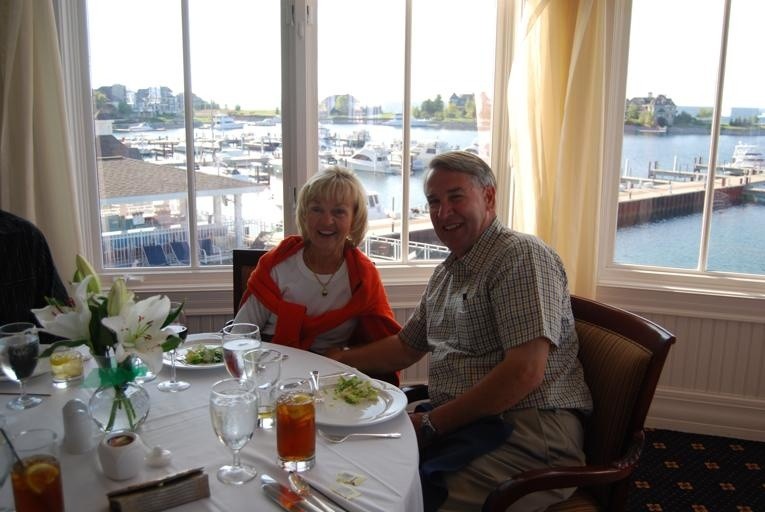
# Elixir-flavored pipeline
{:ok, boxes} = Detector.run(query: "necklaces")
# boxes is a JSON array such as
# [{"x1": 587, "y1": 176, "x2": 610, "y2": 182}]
[{"x1": 307, "y1": 250, "x2": 345, "y2": 296}]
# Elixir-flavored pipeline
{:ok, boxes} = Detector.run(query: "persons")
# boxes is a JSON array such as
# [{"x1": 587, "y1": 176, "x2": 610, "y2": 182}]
[
  {"x1": 331, "y1": 151, "x2": 597, "y2": 511},
  {"x1": 1, "y1": 208, "x2": 74, "y2": 344},
  {"x1": 231, "y1": 165, "x2": 407, "y2": 388}
]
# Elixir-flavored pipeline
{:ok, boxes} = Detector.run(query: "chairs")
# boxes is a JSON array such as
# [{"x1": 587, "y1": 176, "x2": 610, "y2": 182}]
[
  {"x1": 401, "y1": 295, "x2": 675, "y2": 510},
  {"x1": 250, "y1": 231, "x2": 274, "y2": 248},
  {"x1": 142, "y1": 239, "x2": 233, "y2": 266},
  {"x1": 233, "y1": 249, "x2": 266, "y2": 319}
]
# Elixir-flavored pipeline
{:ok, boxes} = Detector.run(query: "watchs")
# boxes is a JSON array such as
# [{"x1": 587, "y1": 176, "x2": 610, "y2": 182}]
[{"x1": 421, "y1": 412, "x2": 437, "y2": 440}]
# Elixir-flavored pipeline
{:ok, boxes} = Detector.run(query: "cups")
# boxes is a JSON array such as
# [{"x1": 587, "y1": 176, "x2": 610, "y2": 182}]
[
  {"x1": 221, "y1": 322, "x2": 262, "y2": 380},
  {"x1": 242, "y1": 348, "x2": 284, "y2": 415},
  {"x1": 97, "y1": 429, "x2": 142, "y2": 482},
  {"x1": 10, "y1": 452, "x2": 66, "y2": 512},
  {"x1": 276, "y1": 375, "x2": 316, "y2": 474},
  {"x1": 50, "y1": 345, "x2": 84, "y2": 391}
]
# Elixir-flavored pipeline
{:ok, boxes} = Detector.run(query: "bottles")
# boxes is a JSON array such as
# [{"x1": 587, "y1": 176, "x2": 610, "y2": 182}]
[{"x1": 62, "y1": 396, "x2": 94, "y2": 455}]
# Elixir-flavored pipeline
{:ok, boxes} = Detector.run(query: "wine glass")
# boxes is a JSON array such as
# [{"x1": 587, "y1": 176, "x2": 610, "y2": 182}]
[
  {"x1": 156, "y1": 302, "x2": 191, "y2": 392},
  {"x1": 208, "y1": 378, "x2": 259, "y2": 486},
  {"x1": 0, "y1": 322, "x2": 44, "y2": 412}
]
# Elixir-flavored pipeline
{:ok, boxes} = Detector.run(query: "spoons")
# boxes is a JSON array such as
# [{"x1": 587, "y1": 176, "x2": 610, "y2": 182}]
[
  {"x1": 317, "y1": 428, "x2": 402, "y2": 444},
  {"x1": 288, "y1": 473, "x2": 335, "y2": 512}
]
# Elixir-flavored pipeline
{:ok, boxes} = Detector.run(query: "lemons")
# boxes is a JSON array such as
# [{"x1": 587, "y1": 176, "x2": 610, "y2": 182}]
[{"x1": 26, "y1": 463, "x2": 58, "y2": 496}]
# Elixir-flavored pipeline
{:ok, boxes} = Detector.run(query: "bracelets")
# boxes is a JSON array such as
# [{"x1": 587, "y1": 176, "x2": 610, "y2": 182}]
[{"x1": 340, "y1": 346, "x2": 351, "y2": 352}]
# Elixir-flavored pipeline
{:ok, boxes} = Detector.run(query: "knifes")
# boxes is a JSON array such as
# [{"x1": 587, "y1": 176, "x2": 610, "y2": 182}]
[{"x1": 260, "y1": 474, "x2": 324, "y2": 512}]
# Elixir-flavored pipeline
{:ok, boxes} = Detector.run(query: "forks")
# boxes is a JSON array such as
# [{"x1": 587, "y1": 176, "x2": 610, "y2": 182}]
[{"x1": 310, "y1": 369, "x2": 326, "y2": 406}]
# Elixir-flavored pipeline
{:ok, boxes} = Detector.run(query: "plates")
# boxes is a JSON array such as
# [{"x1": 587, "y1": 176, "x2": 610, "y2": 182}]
[
  {"x1": 309, "y1": 373, "x2": 409, "y2": 428},
  {"x1": 163, "y1": 332, "x2": 225, "y2": 370}
]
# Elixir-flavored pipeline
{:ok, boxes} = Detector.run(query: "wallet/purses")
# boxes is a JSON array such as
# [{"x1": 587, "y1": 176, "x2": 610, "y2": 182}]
[{"x1": 106, "y1": 465, "x2": 210, "y2": 512}]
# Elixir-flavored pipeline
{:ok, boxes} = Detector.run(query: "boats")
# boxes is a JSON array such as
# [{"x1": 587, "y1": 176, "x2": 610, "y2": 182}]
[
  {"x1": 724, "y1": 142, "x2": 765, "y2": 176},
  {"x1": 364, "y1": 191, "x2": 389, "y2": 220}
]
